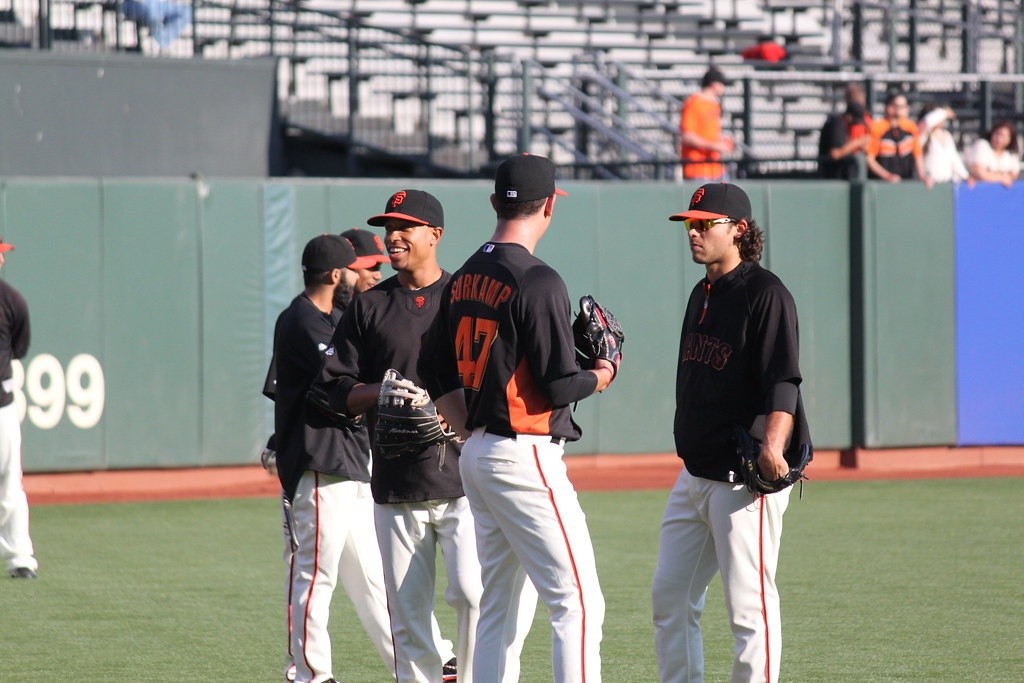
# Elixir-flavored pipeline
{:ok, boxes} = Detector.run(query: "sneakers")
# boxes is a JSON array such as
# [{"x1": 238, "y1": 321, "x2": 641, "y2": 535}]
[{"x1": 438, "y1": 657, "x2": 456, "y2": 681}]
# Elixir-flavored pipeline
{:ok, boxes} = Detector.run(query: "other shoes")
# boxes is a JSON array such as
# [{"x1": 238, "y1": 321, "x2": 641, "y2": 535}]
[{"x1": 10, "y1": 566, "x2": 34, "y2": 578}]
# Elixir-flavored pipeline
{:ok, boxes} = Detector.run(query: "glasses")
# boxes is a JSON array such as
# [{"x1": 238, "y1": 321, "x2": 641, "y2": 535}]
[{"x1": 686, "y1": 218, "x2": 736, "y2": 229}]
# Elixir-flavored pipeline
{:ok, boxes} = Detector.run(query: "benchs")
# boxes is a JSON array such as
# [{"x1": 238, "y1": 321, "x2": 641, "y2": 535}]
[{"x1": 10, "y1": 0, "x2": 1022, "y2": 179}]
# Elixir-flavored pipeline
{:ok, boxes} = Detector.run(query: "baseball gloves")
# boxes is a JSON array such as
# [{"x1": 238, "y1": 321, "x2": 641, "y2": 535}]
[
  {"x1": 570, "y1": 293, "x2": 625, "y2": 389},
  {"x1": 261, "y1": 432, "x2": 278, "y2": 472},
  {"x1": 373, "y1": 368, "x2": 466, "y2": 473},
  {"x1": 731, "y1": 425, "x2": 812, "y2": 501}
]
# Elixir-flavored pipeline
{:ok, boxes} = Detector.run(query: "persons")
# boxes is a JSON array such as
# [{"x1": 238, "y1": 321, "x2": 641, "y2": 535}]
[
  {"x1": 0, "y1": 233, "x2": 38, "y2": 576},
  {"x1": 105, "y1": 0, "x2": 190, "y2": 52},
  {"x1": 313, "y1": 192, "x2": 473, "y2": 683},
  {"x1": 652, "y1": 179, "x2": 803, "y2": 682},
  {"x1": 682, "y1": 64, "x2": 732, "y2": 179},
  {"x1": 864, "y1": 93, "x2": 932, "y2": 189},
  {"x1": 916, "y1": 101, "x2": 976, "y2": 189},
  {"x1": 964, "y1": 120, "x2": 1021, "y2": 189},
  {"x1": 845, "y1": 79, "x2": 873, "y2": 155},
  {"x1": 418, "y1": 152, "x2": 615, "y2": 682},
  {"x1": 262, "y1": 227, "x2": 459, "y2": 683},
  {"x1": 818, "y1": 102, "x2": 870, "y2": 180}
]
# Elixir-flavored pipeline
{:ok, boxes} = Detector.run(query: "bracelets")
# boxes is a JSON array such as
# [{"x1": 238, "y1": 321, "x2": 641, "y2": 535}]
[{"x1": 600, "y1": 367, "x2": 613, "y2": 384}]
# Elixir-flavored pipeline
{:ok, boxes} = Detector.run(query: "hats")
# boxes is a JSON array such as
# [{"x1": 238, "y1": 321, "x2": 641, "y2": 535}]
[
  {"x1": 301, "y1": 233, "x2": 377, "y2": 274},
  {"x1": 0, "y1": 237, "x2": 14, "y2": 253},
  {"x1": 496, "y1": 154, "x2": 568, "y2": 200},
  {"x1": 364, "y1": 190, "x2": 444, "y2": 226},
  {"x1": 701, "y1": 68, "x2": 736, "y2": 88},
  {"x1": 666, "y1": 182, "x2": 752, "y2": 220},
  {"x1": 336, "y1": 228, "x2": 391, "y2": 270}
]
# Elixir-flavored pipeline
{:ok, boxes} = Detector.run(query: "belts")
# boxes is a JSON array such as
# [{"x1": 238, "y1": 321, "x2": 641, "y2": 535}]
[{"x1": 485, "y1": 426, "x2": 560, "y2": 445}]
